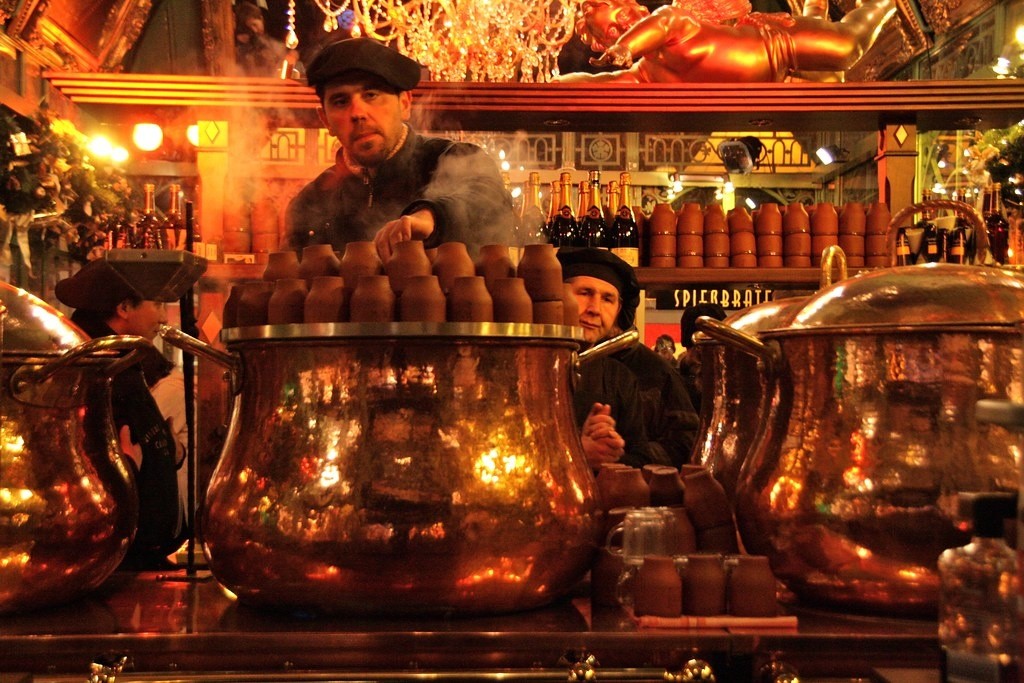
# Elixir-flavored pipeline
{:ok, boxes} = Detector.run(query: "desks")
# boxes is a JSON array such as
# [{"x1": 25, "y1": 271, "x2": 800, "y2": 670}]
[{"x1": 0, "y1": 572, "x2": 947, "y2": 683}]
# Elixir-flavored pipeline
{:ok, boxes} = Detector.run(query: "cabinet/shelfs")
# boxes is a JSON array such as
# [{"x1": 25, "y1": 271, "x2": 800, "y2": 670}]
[{"x1": 44, "y1": 71, "x2": 1024, "y2": 289}]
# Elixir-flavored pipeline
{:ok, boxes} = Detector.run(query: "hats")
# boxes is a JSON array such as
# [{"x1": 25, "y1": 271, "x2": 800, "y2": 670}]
[
  {"x1": 305, "y1": 36, "x2": 423, "y2": 91},
  {"x1": 679, "y1": 302, "x2": 728, "y2": 348},
  {"x1": 555, "y1": 246, "x2": 640, "y2": 300},
  {"x1": 56, "y1": 258, "x2": 131, "y2": 308}
]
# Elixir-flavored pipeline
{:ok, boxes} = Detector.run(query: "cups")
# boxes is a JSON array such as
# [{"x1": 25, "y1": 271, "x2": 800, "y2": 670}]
[
  {"x1": 219, "y1": 241, "x2": 579, "y2": 330},
  {"x1": 595, "y1": 459, "x2": 777, "y2": 617},
  {"x1": 629, "y1": 203, "x2": 890, "y2": 268}
]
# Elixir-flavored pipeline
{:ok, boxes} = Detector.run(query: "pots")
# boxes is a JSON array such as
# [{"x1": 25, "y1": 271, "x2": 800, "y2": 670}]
[
  {"x1": 693, "y1": 199, "x2": 1024, "y2": 617},
  {"x1": 1, "y1": 278, "x2": 155, "y2": 621},
  {"x1": 155, "y1": 321, "x2": 638, "y2": 618}
]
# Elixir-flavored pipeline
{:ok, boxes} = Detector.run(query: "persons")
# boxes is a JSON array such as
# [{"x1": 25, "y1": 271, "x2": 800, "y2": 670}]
[
  {"x1": 235, "y1": 1, "x2": 307, "y2": 78},
  {"x1": 549, "y1": 0, "x2": 897, "y2": 83},
  {"x1": 556, "y1": 247, "x2": 701, "y2": 469},
  {"x1": 54, "y1": 257, "x2": 189, "y2": 571},
  {"x1": 678, "y1": 302, "x2": 726, "y2": 419},
  {"x1": 653, "y1": 334, "x2": 677, "y2": 368},
  {"x1": 283, "y1": 39, "x2": 509, "y2": 275}
]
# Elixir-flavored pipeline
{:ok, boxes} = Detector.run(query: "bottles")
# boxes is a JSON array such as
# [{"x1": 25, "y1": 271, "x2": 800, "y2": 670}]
[
  {"x1": 503, "y1": 171, "x2": 639, "y2": 267},
  {"x1": 923, "y1": 182, "x2": 1008, "y2": 267},
  {"x1": 101, "y1": 179, "x2": 186, "y2": 251}
]
